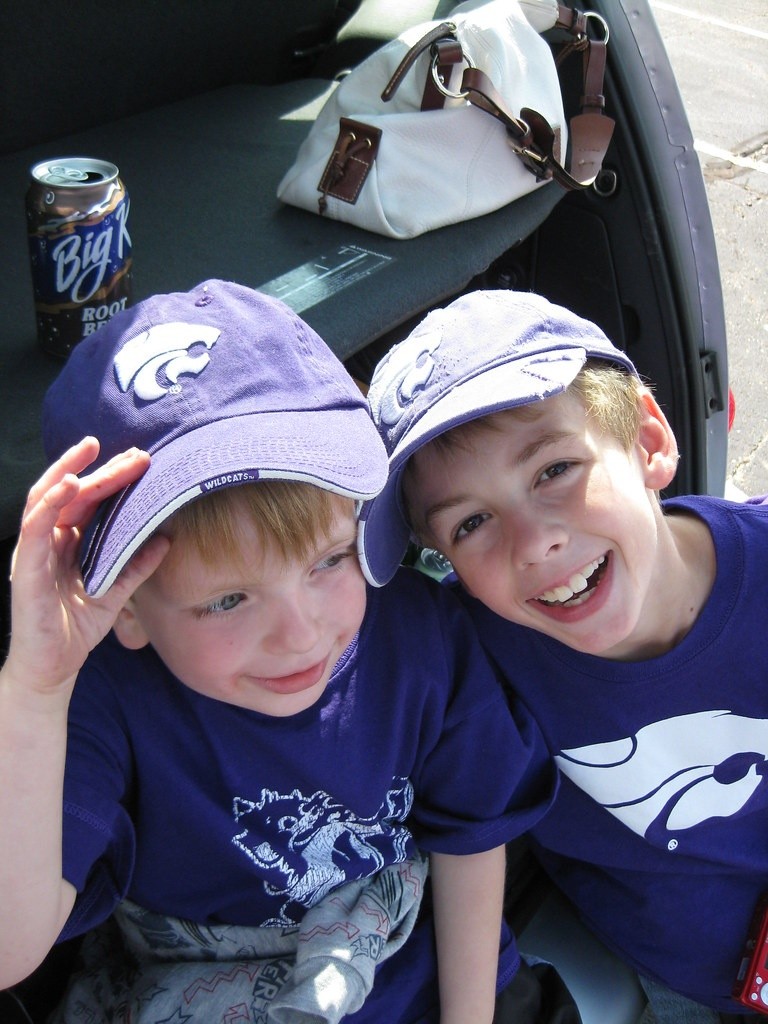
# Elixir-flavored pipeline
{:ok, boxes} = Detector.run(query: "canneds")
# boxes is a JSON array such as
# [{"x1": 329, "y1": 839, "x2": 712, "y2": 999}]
[{"x1": 26, "y1": 156, "x2": 131, "y2": 358}]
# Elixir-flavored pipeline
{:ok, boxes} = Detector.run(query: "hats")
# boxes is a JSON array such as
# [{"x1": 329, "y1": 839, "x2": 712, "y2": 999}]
[
  {"x1": 46, "y1": 279, "x2": 390, "y2": 599},
  {"x1": 355, "y1": 290, "x2": 642, "y2": 593}
]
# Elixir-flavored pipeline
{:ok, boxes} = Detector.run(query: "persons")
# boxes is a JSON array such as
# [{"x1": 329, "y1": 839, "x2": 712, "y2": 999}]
[
  {"x1": 0, "y1": 279, "x2": 561, "y2": 1024},
  {"x1": 358, "y1": 288, "x2": 768, "y2": 1024}
]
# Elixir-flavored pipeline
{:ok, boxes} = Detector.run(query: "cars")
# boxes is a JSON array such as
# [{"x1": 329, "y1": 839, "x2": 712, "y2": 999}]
[{"x1": 1, "y1": 1, "x2": 738, "y2": 1024}]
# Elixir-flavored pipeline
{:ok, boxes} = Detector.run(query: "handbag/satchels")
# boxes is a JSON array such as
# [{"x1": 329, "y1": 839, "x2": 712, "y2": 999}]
[{"x1": 276, "y1": 0, "x2": 615, "y2": 239}]
